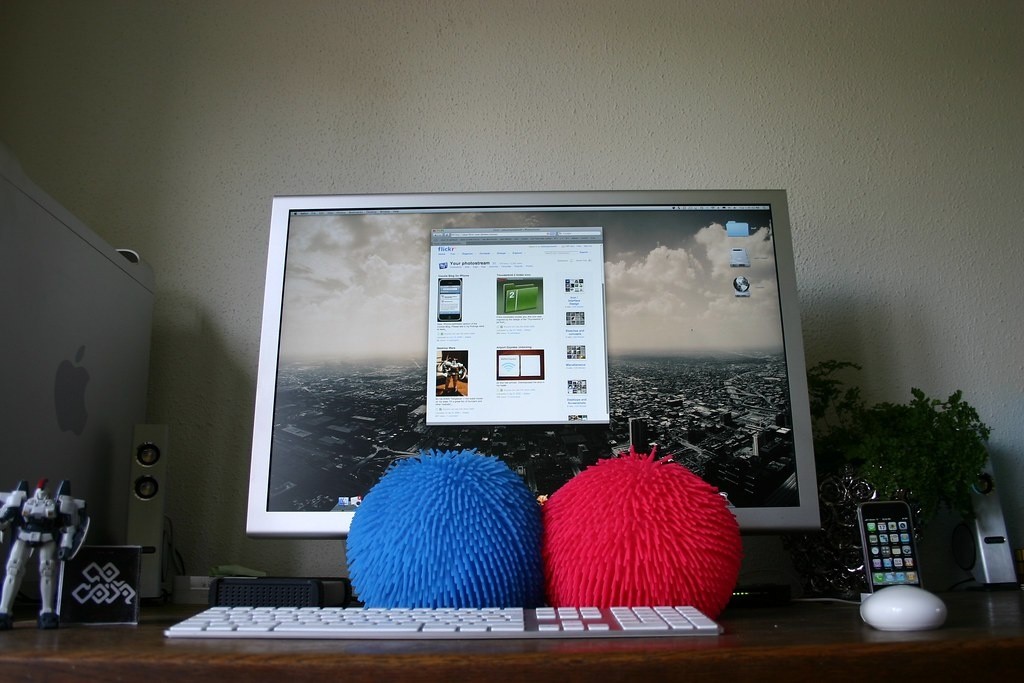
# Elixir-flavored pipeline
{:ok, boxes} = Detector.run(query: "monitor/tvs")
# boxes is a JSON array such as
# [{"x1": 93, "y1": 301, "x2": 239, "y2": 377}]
[{"x1": 246, "y1": 188, "x2": 820, "y2": 539}]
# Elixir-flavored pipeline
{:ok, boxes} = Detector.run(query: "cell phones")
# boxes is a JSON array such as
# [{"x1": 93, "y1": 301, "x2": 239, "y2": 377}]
[
  {"x1": 858, "y1": 501, "x2": 923, "y2": 594},
  {"x1": 438, "y1": 279, "x2": 461, "y2": 322}
]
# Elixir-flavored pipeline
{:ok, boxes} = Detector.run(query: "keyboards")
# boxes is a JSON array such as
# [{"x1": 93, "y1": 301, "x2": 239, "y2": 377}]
[{"x1": 162, "y1": 605, "x2": 725, "y2": 638}]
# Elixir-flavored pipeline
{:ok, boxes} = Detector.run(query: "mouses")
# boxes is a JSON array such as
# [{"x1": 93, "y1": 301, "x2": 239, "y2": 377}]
[{"x1": 860, "y1": 584, "x2": 947, "y2": 631}]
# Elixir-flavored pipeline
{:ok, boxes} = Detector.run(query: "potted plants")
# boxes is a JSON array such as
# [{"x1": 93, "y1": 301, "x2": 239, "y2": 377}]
[{"x1": 783, "y1": 360, "x2": 989, "y2": 603}]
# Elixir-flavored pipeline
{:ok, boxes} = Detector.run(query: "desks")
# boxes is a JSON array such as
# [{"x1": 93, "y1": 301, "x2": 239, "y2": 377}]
[{"x1": 0, "y1": 604, "x2": 1024, "y2": 683}]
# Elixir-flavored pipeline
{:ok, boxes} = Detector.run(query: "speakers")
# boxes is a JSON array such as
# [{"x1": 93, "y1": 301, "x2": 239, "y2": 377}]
[
  {"x1": 949, "y1": 437, "x2": 1018, "y2": 590},
  {"x1": 123, "y1": 421, "x2": 169, "y2": 599}
]
